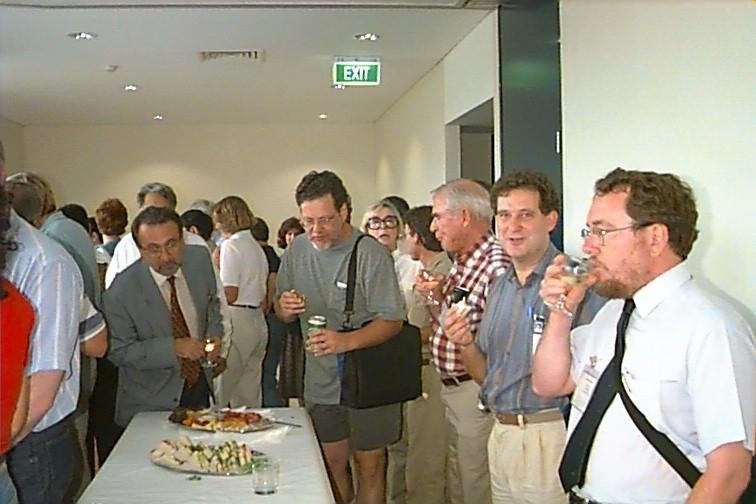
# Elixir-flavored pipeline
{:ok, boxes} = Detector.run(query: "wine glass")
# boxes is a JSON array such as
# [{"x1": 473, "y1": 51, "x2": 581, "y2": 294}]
[
  {"x1": 540, "y1": 250, "x2": 603, "y2": 313},
  {"x1": 421, "y1": 262, "x2": 443, "y2": 305},
  {"x1": 200, "y1": 334, "x2": 218, "y2": 367}
]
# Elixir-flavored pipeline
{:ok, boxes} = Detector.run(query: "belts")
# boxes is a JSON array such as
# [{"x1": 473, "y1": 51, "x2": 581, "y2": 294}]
[
  {"x1": 230, "y1": 304, "x2": 257, "y2": 309},
  {"x1": 497, "y1": 410, "x2": 563, "y2": 424},
  {"x1": 442, "y1": 373, "x2": 471, "y2": 385}
]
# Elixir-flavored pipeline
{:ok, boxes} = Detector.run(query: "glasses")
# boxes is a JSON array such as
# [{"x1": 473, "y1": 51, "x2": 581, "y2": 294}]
[
  {"x1": 581, "y1": 226, "x2": 629, "y2": 246},
  {"x1": 368, "y1": 216, "x2": 399, "y2": 230}
]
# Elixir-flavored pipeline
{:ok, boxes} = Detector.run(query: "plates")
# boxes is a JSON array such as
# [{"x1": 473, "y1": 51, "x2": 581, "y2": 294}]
[
  {"x1": 150, "y1": 447, "x2": 266, "y2": 474},
  {"x1": 171, "y1": 410, "x2": 273, "y2": 432}
]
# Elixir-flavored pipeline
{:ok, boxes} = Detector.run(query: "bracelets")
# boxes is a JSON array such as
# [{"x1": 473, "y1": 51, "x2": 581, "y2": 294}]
[{"x1": 458, "y1": 338, "x2": 475, "y2": 347}]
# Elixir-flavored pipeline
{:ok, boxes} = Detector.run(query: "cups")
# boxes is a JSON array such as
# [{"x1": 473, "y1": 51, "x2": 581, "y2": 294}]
[{"x1": 251, "y1": 455, "x2": 279, "y2": 493}]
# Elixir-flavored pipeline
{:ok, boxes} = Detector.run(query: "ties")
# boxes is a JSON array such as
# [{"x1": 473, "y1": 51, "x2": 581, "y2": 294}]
[
  {"x1": 558, "y1": 297, "x2": 636, "y2": 493},
  {"x1": 167, "y1": 276, "x2": 199, "y2": 388}
]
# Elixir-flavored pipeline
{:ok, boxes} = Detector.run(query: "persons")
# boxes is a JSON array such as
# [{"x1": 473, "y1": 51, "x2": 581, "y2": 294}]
[
  {"x1": 1, "y1": 137, "x2": 512, "y2": 503},
  {"x1": 531, "y1": 166, "x2": 754, "y2": 503},
  {"x1": 443, "y1": 168, "x2": 610, "y2": 503}
]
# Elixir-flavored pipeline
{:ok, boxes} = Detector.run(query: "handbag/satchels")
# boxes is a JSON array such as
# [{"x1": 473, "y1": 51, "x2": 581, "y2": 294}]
[{"x1": 339, "y1": 319, "x2": 422, "y2": 408}]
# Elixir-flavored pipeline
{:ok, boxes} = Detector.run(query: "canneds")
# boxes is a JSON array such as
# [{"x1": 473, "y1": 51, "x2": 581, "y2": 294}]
[{"x1": 305, "y1": 316, "x2": 327, "y2": 352}]
